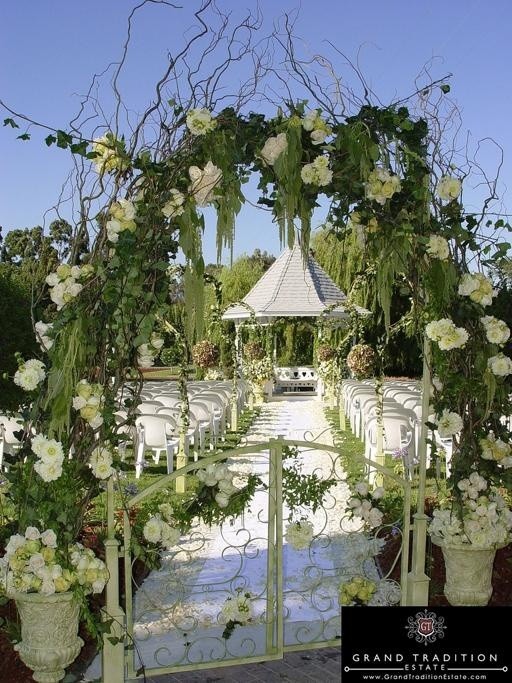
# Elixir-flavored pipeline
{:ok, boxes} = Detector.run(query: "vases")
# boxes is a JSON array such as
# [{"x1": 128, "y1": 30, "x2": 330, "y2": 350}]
[
  {"x1": 431, "y1": 535, "x2": 510, "y2": 606},
  {"x1": 3, "y1": 590, "x2": 94, "y2": 683}
]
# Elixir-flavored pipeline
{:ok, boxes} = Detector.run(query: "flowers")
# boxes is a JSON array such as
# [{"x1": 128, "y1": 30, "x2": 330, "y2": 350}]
[{"x1": 0, "y1": 76, "x2": 512, "y2": 594}]
